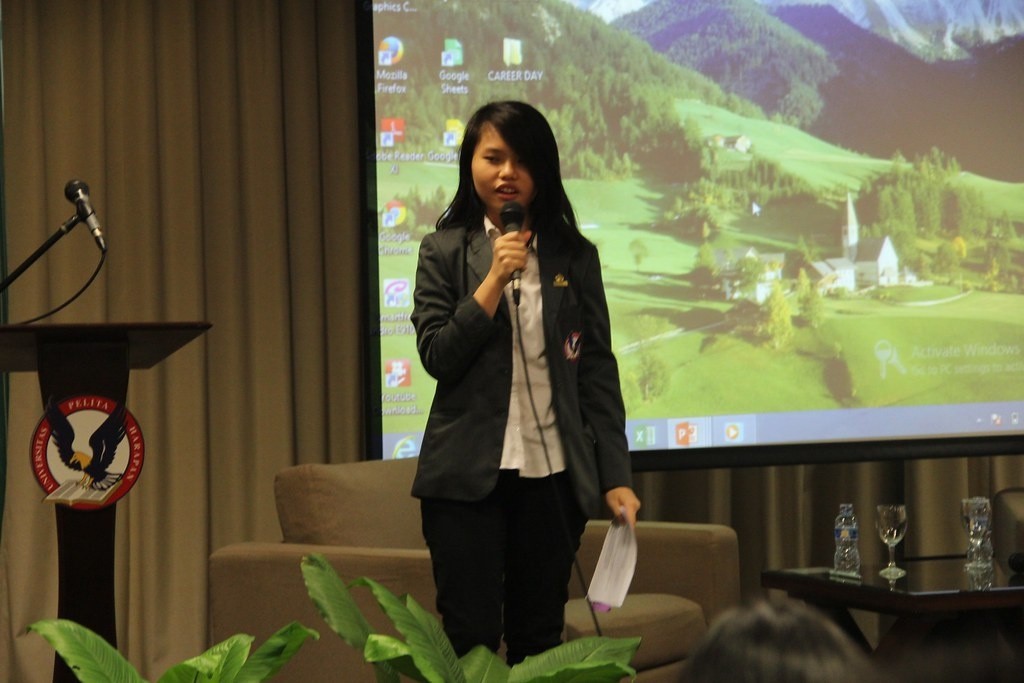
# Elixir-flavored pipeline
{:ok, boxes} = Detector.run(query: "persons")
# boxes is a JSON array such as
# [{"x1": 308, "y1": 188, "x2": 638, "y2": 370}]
[{"x1": 409, "y1": 101, "x2": 641, "y2": 666}]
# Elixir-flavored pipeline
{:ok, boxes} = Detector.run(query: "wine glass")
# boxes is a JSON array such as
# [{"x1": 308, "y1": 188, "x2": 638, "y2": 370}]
[
  {"x1": 875, "y1": 504, "x2": 907, "y2": 579},
  {"x1": 960, "y1": 497, "x2": 991, "y2": 574}
]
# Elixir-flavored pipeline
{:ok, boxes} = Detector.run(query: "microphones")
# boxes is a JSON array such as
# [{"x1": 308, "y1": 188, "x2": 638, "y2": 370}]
[
  {"x1": 499, "y1": 201, "x2": 525, "y2": 306},
  {"x1": 64, "y1": 179, "x2": 106, "y2": 253}
]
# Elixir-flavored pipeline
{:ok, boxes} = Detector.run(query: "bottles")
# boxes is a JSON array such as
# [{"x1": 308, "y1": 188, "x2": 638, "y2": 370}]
[{"x1": 834, "y1": 504, "x2": 860, "y2": 573}]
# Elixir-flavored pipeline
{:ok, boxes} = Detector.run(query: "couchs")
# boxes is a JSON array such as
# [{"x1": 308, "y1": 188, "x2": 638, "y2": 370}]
[{"x1": 207, "y1": 455, "x2": 743, "y2": 683}]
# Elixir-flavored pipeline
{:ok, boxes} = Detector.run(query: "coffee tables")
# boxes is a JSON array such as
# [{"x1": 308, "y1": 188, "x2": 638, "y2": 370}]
[{"x1": 762, "y1": 557, "x2": 1024, "y2": 667}]
